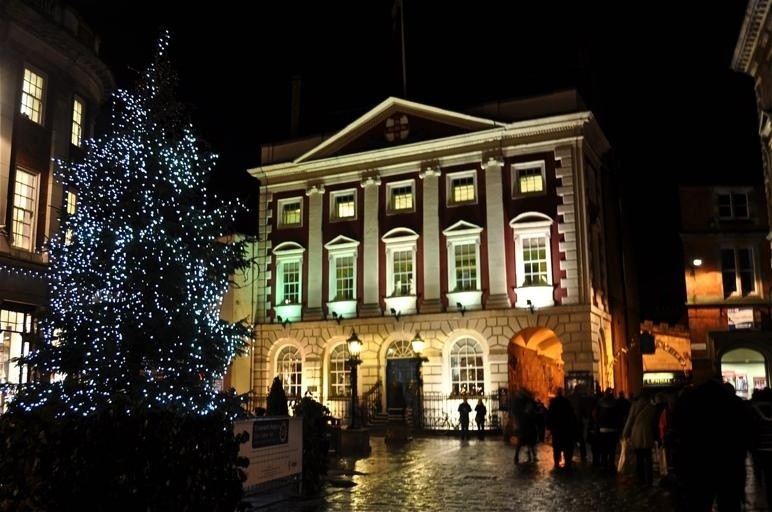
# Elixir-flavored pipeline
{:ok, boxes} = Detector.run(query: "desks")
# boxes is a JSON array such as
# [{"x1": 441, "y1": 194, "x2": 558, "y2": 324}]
[
  {"x1": 345, "y1": 327, "x2": 366, "y2": 431},
  {"x1": 410, "y1": 328, "x2": 430, "y2": 437}
]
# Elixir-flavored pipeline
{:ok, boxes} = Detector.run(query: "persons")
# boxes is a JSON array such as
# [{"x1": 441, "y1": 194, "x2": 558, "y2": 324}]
[
  {"x1": 475, "y1": 397, "x2": 486, "y2": 439},
  {"x1": 458, "y1": 395, "x2": 469, "y2": 442},
  {"x1": 504, "y1": 367, "x2": 771, "y2": 511}
]
